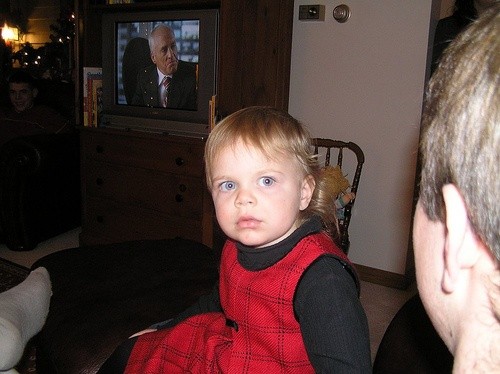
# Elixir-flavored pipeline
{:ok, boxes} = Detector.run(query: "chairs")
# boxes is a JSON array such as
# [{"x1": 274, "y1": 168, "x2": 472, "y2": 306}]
[{"x1": 310, "y1": 137, "x2": 364, "y2": 253}]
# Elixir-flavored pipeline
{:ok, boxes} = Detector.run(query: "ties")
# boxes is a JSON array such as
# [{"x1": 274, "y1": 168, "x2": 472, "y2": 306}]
[{"x1": 163, "y1": 76, "x2": 171, "y2": 107}]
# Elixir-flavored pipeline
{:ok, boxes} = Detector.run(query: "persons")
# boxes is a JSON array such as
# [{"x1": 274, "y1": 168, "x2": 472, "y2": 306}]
[
  {"x1": 409, "y1": 6, "x2": 500, "y2": 374},
  {"x1": 131, "y1": 23, "x2": 197, "y2": 110},
  {"x1": 0, "y1": 265, "x2": 53, "y2": 374},
  {"x1": 114, "y1": 105, "x2": 373, "y2": 374}
]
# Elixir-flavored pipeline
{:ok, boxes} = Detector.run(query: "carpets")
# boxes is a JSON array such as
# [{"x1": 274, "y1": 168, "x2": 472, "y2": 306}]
[{"x1": 0, "y1": 257, "x2": 38, "y2": 374}]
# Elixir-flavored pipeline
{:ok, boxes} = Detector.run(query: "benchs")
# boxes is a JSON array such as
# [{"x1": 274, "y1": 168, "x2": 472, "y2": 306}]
[{"x1": 29, "y1": 238, "x2": 219, "y2": 374}]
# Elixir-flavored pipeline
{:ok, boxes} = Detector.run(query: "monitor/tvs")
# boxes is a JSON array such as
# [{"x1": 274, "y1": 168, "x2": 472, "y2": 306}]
[{"x1": 101, "y1": 9, "x2": 218, "y2": 140}]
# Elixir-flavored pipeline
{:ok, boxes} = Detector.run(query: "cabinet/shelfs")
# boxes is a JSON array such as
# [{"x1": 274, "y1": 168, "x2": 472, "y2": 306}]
[{"x1": 78, "y1": 0, "x2": 294, "y2": 249}]
